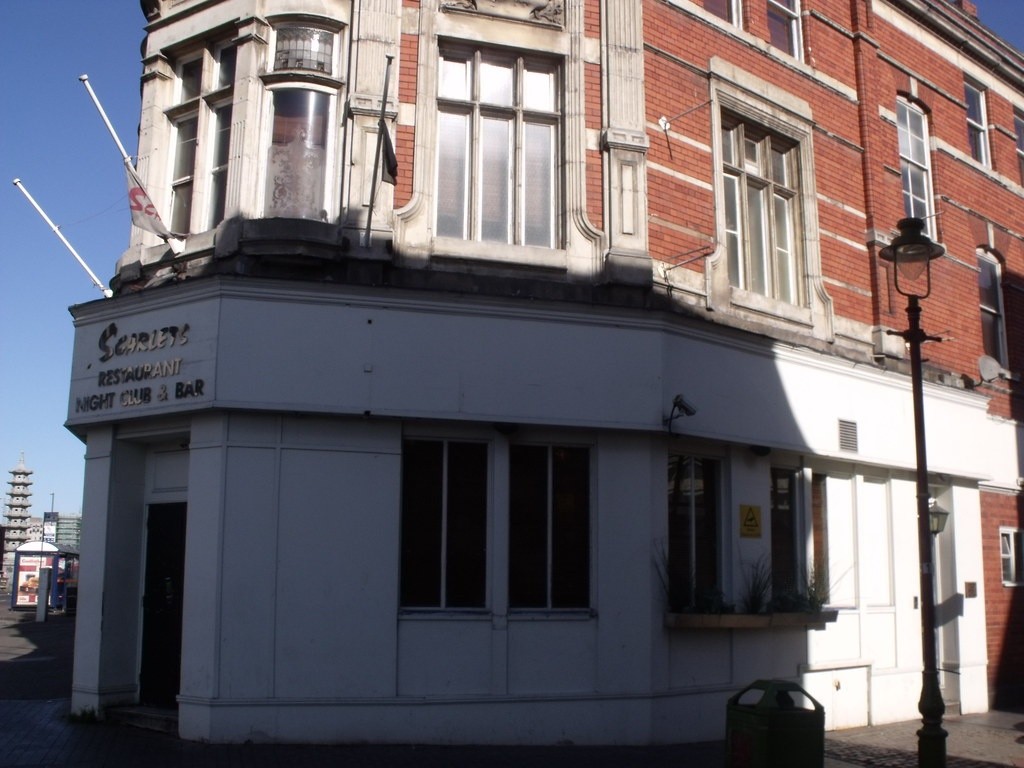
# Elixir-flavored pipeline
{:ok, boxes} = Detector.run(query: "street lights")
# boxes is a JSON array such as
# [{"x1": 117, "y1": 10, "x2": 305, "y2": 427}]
[{"x1": 878, "y1": 216, "x2": 948, "y2": 768}]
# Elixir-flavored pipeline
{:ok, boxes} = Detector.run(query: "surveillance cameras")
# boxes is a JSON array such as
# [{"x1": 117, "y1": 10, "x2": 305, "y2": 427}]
[{"x1": 673, "y1": 393, "x2": 697, "y2": 416}]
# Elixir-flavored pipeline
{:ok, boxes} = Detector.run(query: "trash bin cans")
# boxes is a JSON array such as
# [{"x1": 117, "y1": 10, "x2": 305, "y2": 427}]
[{"x1": 727, "y1": 677, "x2": 825, "y2": 768}]
